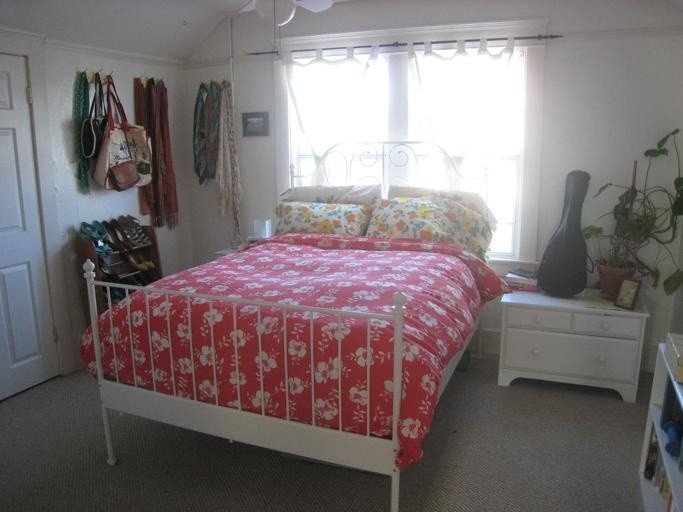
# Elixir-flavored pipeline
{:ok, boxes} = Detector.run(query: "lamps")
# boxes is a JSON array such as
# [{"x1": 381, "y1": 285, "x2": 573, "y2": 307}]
[{"x1": 214, "y1": 0, "x2": 334, "y2": 27}]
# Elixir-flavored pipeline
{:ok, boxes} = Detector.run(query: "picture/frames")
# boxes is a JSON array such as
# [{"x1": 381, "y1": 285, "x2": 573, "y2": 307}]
[{"x1": 243, "y1": 112, "x2": 269, "y2": 136}]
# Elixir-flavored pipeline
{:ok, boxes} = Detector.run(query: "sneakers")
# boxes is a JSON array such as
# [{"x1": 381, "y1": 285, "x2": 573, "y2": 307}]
[{"x1": 101, "y1": 269, "x2": 162, "y2": 299}]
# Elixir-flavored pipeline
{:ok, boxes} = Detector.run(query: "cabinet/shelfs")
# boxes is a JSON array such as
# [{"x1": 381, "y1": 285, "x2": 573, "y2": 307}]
[
  {"x1": 496, "y1": 289, "x2": 650, "y2": 402},
  {"x1": 639, "y1": 342, "x2": 683, "y2": 512},
  {"x1": 76, "y1": 225, "x2": 164, "y2": 328}
]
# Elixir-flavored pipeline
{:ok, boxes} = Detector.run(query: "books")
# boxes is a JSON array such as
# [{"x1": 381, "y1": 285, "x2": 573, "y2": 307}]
[
  {"x1": 643, "y1": 331, "x2": 683, "y2": 512},
  {"x1": 504, "y1": 267, "x2": 539, "y2": 290}
]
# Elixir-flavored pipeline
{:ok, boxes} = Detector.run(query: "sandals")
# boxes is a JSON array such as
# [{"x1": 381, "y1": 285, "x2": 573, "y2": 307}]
[{"x1": 81, "y1": 215, "x2": 152, "y2": 255}]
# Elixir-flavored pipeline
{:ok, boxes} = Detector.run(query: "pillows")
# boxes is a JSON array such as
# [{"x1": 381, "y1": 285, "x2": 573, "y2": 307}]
[{"x1": 272, "y1": 182, "x2": 496, "y2": 253}]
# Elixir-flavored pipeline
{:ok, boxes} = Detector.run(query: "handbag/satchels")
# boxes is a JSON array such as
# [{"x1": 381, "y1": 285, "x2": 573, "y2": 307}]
[
  {"x1": 93, "y1": 122, "x2": 153, "y2": 191},
  {"x1": 81, "y1": 116, "x2": 108, "y2": 158}
]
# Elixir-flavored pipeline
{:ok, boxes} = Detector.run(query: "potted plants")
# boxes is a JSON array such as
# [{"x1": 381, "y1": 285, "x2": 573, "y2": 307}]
[{"x1": 577, "y1": 157, "x2": 678, "y2": 300}]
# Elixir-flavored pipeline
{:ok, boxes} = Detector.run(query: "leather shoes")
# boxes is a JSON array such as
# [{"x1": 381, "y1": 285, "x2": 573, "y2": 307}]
[
  {"x1": 119, "y1": 248, "x2": 156, "y2": 272},
  {"x1": 99, "y1": 257, "x2": 120, "y2": 280}
]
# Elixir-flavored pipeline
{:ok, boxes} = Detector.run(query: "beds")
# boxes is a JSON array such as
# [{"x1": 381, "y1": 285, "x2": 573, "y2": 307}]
[{"x1": 80, "y1": 141, "x2": 485, "y2": 512}]
